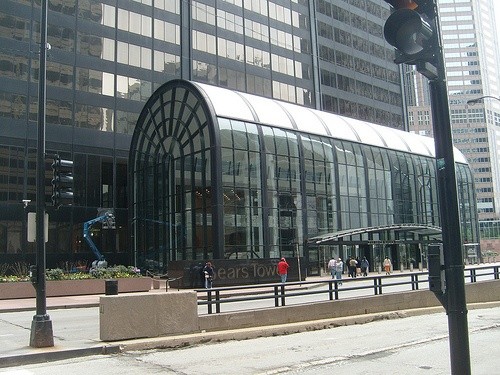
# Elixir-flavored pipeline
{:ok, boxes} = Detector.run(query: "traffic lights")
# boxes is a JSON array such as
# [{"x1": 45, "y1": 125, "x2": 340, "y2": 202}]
[
  {"x1": 50, "y1": 159, "x2": 75, "y2": 207},
  {"x1": 385, "y1": 0, "x2": 442, "y2": 80}
]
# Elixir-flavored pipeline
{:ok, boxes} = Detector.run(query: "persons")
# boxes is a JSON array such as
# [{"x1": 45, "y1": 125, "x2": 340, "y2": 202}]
[
  {"x1": 355, "y1": 257, "x2": 362, "y2": 276},
  {"x1": 346, "y1": 255, "x2": 352, "y2": 277},
  {"x1": 383, "y1": 256, "x2": 392, "y2": 275},
  {"x1": 349, "y1": 257, "x2": 358, "y2": 277},
  {"x1": 336, "y1": 257, "x2": 343, "y2": 286},
  {"x1": 277, "y1": 257, "x2": 289, "y2": 283},
  {"x1": 361, "y1": 257, "x2": 369, "y2": 276},
  {"x1": 203, "y1": 263, "x2": 213, "y2": 289},
  {"x1": 328, "y1": 257, "x2": 337, "y2": 279}
]
